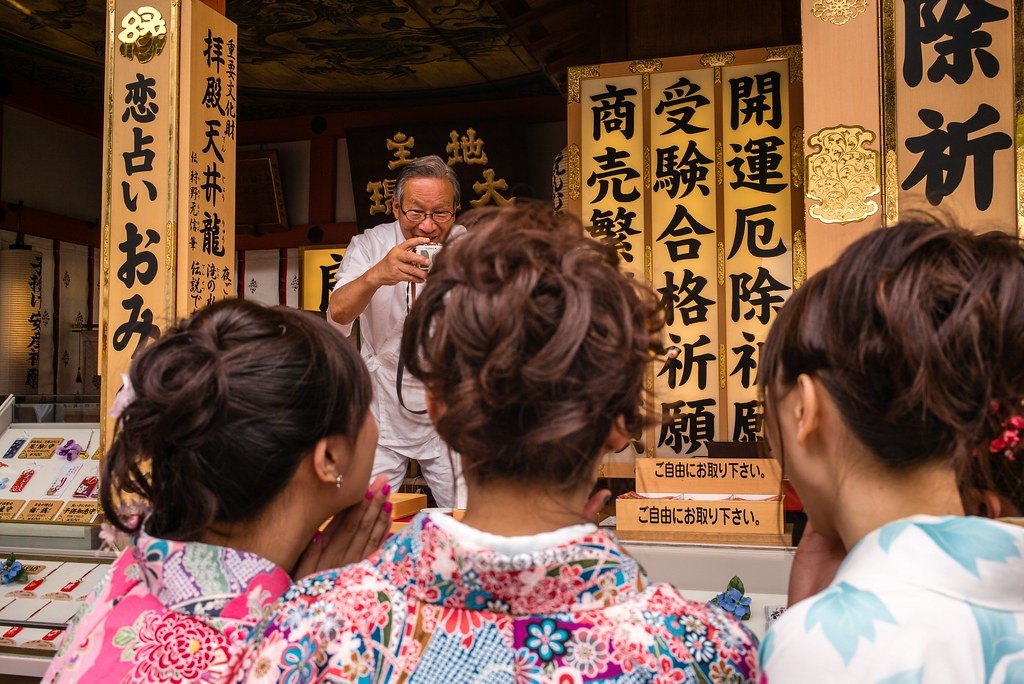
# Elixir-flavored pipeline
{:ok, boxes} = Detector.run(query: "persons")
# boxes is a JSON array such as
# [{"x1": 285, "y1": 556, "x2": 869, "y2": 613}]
[
  {"x1": 224, "y1": 198, "x2": 769, "y2": 684},
  {"x1": 323, "y1": 156, "x2": 469, "y2": 510},
  {"x1": 753, "y1": 203, "x2": 1024, "y2": 684},
  {"x1": 38, "y1": 294, "x2": 397, "y2": 684}
]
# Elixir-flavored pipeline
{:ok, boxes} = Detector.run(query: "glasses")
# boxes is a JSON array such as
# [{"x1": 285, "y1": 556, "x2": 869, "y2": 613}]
[{"x1": 400, "y1": 206, "x2": 455, "y2": 223}]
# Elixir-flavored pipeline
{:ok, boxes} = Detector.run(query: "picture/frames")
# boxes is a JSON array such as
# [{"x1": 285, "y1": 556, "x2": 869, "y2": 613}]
[{"x1": 235, "y1": 151, "x2": 291, "y2": 235}]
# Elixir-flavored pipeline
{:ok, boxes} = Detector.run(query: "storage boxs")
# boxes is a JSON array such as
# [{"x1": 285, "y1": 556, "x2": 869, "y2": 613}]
[
  {"x1": 615, "y1": 493, "x2": 786, "y2": 535},
  {"x1": 389, "y1": 493, "x2": 427, "y2": 521}
]
